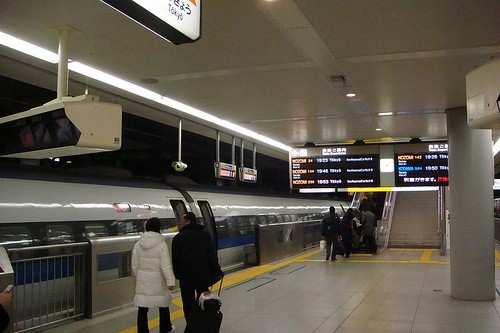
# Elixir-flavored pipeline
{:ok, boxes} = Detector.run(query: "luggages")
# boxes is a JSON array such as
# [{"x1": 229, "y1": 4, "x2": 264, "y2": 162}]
[{"x1": 184, "y1": 276, "x2": 224, "y2": 333}]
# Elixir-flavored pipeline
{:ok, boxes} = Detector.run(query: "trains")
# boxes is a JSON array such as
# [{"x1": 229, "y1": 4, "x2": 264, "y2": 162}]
[{"x1": 0, "y1": 177, "x2": 360, "y2": 331}]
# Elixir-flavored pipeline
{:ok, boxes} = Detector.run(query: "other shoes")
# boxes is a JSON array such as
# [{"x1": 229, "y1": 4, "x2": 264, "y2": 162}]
[
  {"x1": 168, "y1": 325, "x2": 175, "y2": 333},
  {"x1": 326, "y1": 258, "x2": 329, "y2": 260},
  {"x1": 332, "y1": 259, "x2": 336, "y2": 261}
]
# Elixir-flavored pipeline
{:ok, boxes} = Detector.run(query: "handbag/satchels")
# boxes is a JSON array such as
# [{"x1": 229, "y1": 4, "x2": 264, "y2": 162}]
[{"x1": 320, "y1": 235, "x2": 326, "y2": 250}]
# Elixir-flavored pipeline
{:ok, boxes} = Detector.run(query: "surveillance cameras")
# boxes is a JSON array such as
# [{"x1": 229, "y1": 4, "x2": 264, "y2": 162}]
[{"x1": 174, "y1": 163, "x2": 185, "y2": 172}]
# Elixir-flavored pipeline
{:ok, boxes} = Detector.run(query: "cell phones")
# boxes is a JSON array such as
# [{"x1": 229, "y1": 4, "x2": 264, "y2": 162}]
[{"x1": 4, "y1": 284, "x2": 14, "y2": 293}]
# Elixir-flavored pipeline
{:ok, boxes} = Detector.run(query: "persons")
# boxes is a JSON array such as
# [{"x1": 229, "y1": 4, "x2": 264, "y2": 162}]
[
  {"x1": 320, "y1": 207, "x2": 341, "y2": 261},
  {"x1": 0, "y1": 290, "x2": 14, "y2": 333},
  {"x1": 131, "y1": 217, "x2": 176, "y2": 333},
  {"x1": 340, "y1": 192, "x2": 386, "y2": 258},
  {"x1": 171, "y1": 212, "x2": 224, "y2": 325}
]
